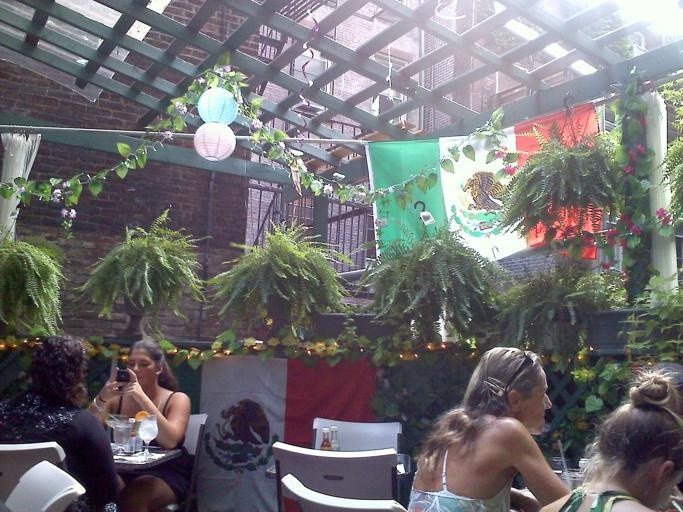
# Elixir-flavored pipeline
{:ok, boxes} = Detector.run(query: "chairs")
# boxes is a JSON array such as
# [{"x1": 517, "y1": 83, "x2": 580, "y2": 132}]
[
  {"x1": 273, "y1": 417, "x2": 408, "y2": 512},
  {"x1": 1, "y1": 412, "x2": 79, "y2": 510},
  {"x1": 161, "y1": 413, "x2": 208, "y2": 511}
]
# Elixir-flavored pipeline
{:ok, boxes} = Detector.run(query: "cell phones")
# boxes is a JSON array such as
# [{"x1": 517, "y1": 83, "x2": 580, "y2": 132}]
[{"x1": 116, "y1": 370, "x2": 130, "y2": 390}]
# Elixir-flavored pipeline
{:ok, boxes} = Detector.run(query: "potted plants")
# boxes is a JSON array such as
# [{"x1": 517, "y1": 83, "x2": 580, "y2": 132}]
[
  {"x1": 209, "y1": 218, "x2": 361, "y2": 341},
  {"x1": 72, "y1": 208, "x2": 218, "y2": 340},
  {"x1": 354, "y1": 214, "x2": 516, "y2": 346},
  {"x1": 487, "y1": 117, "x2": 633, "y2": 242},
  {"x1": 0, "y1": 237, "x2": 69, "y2": 345},
  {"x1": 492, "y1": 254, "x2": 629, "y2": 353}
]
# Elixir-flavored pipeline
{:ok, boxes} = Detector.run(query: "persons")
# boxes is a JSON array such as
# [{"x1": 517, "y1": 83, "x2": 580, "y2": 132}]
[
  {"x1": 0, "y1": 333, "x2": 118, "y2": 512},
  {"x1": 82, "y1": 338, "x2": 194, "y2": 511},
  {"x1": 401, "y1": 344, "x2": 572, "y2": 511},
  {"x1": 537, "y1": 365, "x2": 683, "y2": 512}
]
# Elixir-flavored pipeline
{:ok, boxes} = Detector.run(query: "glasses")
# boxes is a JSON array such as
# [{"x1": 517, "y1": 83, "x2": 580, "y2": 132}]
[{"x1": 504, "y1": 351, "x2": 535, "y2": 394}]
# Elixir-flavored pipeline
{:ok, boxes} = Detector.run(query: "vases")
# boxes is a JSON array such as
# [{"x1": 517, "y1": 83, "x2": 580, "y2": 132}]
[{"x1": 585, "y1": 308, "x2": 659, "y2": 357}]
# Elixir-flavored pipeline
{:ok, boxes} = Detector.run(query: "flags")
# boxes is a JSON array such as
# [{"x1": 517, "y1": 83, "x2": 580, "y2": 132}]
[
  {"x1": 164, "y1": 348, "x2": 385, "y2": 511},
  {"x1": 359, "y1": 109, "x2": 605, "y2": 266}
]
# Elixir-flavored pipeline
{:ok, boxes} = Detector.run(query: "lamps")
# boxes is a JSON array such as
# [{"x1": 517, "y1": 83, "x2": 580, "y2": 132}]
[{"x1": 193, "y1": 86, "x2": 239, "y2": 164}]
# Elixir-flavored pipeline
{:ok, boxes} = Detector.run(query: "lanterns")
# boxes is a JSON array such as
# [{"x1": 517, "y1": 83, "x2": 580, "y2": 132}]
[
  {"x1": 195, "y1": 84, "x2": 240, "y2": 126},
  {"x1": 194, "y1": 124, "x2": 237, "y2": 169}
]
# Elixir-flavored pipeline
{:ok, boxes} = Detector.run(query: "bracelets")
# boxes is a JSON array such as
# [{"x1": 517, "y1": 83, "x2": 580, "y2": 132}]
[
  {"x1": 91, "y1": 395, "x2": 101, "y2": 411},
  {"x1": 98, "y1": 392, "x2": 105, "y2": 402}
]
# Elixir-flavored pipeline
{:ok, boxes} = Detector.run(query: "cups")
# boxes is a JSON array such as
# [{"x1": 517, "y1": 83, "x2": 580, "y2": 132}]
[
  {"x1": 552, "y1": 456, "x2": 588, "y2": 491},
  {"x1": 128, "y1": 417, "x2": 144, "y2": 452}
]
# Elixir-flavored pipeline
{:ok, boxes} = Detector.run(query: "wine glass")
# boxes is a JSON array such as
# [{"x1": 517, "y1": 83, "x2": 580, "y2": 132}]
[
  {"x1": 113, "y1": 420, "x2": 134, "y2": 456},
  {"x1": 138, "y1": 415, "x2": 158, "y2": 461}
]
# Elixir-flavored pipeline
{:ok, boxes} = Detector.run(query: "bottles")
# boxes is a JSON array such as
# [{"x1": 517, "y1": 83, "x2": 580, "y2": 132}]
[{"x1": 321, "y1": 426, "x2": 340, "y2": 451}]
